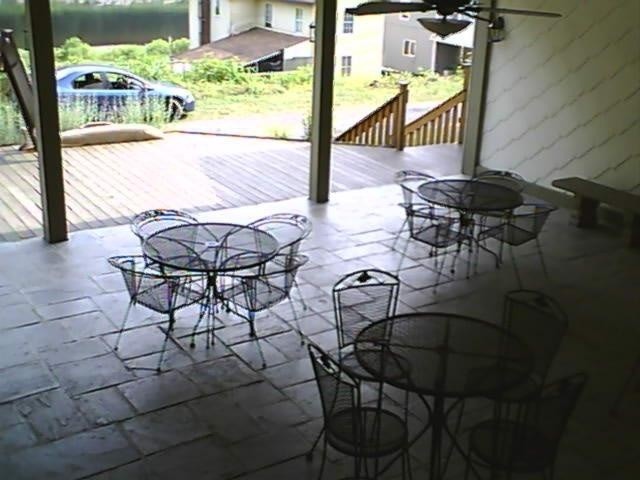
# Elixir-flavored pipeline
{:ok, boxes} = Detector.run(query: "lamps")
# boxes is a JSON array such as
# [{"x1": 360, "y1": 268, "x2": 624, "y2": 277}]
[
  {"x1": 416, "y1": 13, "x2": 472, "y2": 39},
  {"x1": 488, "y1": 10, "x2": 508, "y2": 41}
]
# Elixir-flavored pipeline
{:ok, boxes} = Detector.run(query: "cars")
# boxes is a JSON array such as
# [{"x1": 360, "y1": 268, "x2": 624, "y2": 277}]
[{"x1": 53, "y1": 62, "x2": 196, "y2": 124}]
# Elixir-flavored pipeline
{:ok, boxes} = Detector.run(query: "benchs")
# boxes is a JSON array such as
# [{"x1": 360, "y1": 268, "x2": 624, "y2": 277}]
[{"x1": 551, "y1": 176, "x2": 640, "y2": 242}]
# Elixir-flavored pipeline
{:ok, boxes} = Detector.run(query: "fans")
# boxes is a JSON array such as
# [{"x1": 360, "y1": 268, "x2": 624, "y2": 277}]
[{"x1": 345, "y1": 1, "x2": 562, "y2": 38}]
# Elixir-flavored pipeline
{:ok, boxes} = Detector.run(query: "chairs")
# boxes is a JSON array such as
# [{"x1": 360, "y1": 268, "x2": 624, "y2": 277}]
[
  {"x1": 395, "y1": 168, "x2": 557, "y2": 296},
  {"x1": 107, "y1": 208, "x2": 313, "y2": 375}
]
